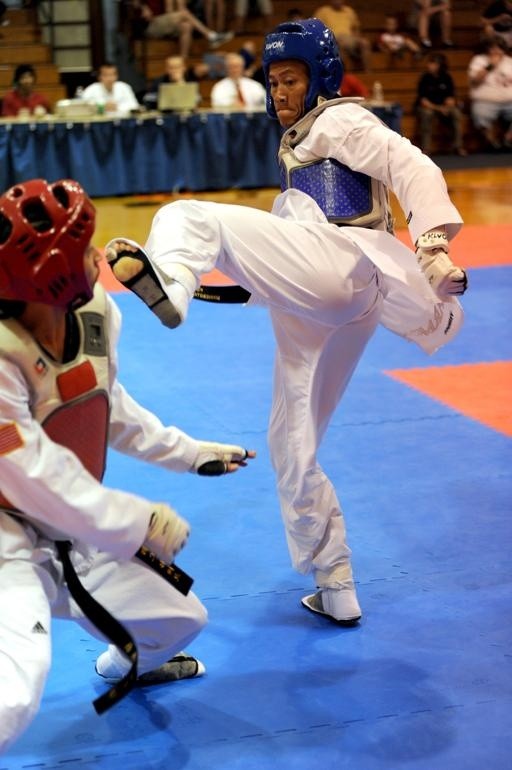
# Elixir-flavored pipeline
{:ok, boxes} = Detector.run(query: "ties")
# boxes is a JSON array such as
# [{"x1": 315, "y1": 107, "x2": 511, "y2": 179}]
[{"x1": 236, "y1": 84, "x2": 243, "y2": 103}]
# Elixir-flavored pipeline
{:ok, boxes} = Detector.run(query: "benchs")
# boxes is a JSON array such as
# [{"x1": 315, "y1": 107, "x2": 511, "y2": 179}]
[{"x1": 0, "y1": 8, "x2": 68, "y2": 109}]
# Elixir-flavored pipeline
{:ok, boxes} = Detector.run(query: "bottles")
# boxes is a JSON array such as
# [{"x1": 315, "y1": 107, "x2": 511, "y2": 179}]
[{"x1": 372, "y1": 80, "x2": 385, "y2": 106}]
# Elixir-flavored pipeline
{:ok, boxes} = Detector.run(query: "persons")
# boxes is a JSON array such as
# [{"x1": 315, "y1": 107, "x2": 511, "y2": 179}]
[
  {"x1": 78, "y1": 61, "x2": 137, "y2": 117},
  {"x1": 415, "y1": 52, "x2": 467, "y2": 157},
  {"x1": 0, "y1": 175, "x2": 260, "y2": 741},
  {"x1": 0, "y1": 60, "x2": 56, "y2": 118},
  {"x1": 123, "y1": 1, "x2": 512, "y2": 79},
  {"x1": 209, "y1": 52, "x2": 267, "y2": 110},
  {"x1": 100, "y1": 14, "x2": 472, "y2": 625},
  {"x1": 467, "y1": 36, "x2": 512, "y2": 153},
  {"x1": 336, "y1": 70, "x2": 369, "y2": 97},
  {"x1": 143, "y1": 52, "x2": 209, "y2": 111}
]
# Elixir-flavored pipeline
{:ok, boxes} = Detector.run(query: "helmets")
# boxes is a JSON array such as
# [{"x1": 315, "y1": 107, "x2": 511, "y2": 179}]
[
  {"x1": 1, "y1": 177, "x2": 97, "y2": 312},
  {"x1": 262, "y1": 18, "x2": 346, "y2": 118}
]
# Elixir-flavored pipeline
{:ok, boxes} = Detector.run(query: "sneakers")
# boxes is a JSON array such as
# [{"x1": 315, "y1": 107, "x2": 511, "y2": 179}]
[{"x1": 456, "y1": 143, "x2": 467, "y2": 156}]
